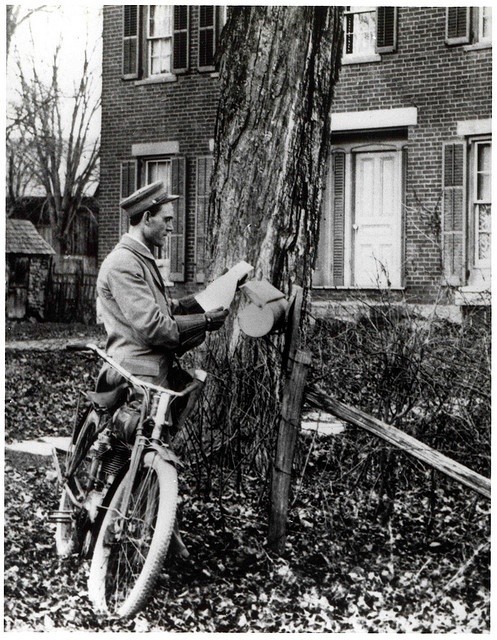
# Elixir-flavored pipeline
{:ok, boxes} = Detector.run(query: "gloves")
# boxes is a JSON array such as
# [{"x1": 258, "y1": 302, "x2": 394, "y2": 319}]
[
  {"x1": 173, "y1": 305, "x2": 231, "y2": 355},
  {"x1": 178, "y1": 266, "x2": 247, "y2": 314}
]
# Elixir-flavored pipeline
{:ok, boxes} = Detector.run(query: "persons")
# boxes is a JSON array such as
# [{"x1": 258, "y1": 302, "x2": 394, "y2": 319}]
[{"x1": 96, "y1": 179, "x2": 248, "y2": 567}]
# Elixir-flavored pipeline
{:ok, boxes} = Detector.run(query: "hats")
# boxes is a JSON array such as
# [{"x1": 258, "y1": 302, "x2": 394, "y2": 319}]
[{"x1": 118, "y1": 181, "x2": 181, "y2": 219}]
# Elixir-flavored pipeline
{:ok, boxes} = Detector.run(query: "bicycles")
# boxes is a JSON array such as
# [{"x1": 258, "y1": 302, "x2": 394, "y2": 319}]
[{"x1": 46, "y1": 342, "x2": 207, "y2": 620}]
